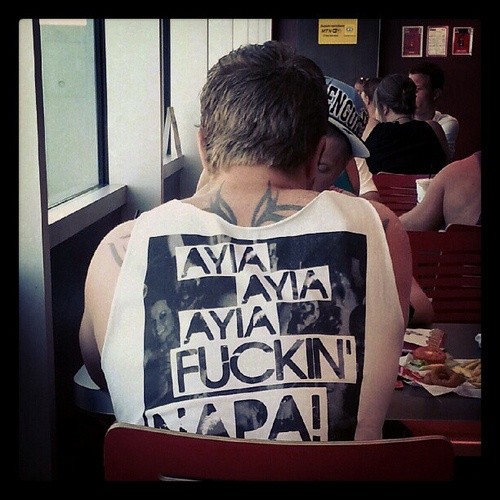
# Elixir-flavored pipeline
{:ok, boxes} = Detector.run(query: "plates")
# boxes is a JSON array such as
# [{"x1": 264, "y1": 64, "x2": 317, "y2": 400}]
[{"x1": 453, "y1": 358, "x2": 481, "y2": 396}]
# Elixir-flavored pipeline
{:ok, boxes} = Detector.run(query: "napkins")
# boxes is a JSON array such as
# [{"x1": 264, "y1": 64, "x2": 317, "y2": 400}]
[
  {"x1": 398, "y1": 352, "x2": 482, "y2": 399},
  {"x1": 403, "y1": 328, "x2": 435, "y2": 347}
]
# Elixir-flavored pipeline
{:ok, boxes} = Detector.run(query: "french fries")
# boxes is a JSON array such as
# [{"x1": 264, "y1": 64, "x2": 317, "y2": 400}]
[{"x1": 419, "y1": 357, "x2": 482, "y2": 387}]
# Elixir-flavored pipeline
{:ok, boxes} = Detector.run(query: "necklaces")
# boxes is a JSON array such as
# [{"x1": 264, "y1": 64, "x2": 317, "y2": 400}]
[{"x1": 394, "y1": 116, "x2": 411, "y2": 124}]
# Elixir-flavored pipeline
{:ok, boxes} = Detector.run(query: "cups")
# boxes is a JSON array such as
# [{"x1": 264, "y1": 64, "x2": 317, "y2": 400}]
[{"x1": 415, "y1": 177, "x2": 432, "y2": 204}]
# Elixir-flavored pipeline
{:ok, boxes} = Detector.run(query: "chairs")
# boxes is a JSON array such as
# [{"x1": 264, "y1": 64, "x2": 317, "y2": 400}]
[
  {"x1": 430, "y1": 218, "x2": 481, "y2": 329},
  {"x1": 374, "y1": 172, "x2": 440, "y2": 220},
  {"x1": 104, "y1": 422, "x2": 459, "y2": 483}
]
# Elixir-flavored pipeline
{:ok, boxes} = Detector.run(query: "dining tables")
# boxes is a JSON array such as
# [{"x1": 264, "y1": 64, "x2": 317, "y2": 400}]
[{"x1": 72, "y1": 321, "x2": 483, "y2": 483}]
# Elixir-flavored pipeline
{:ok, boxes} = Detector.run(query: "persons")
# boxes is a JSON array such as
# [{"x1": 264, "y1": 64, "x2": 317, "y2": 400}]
[
  {"x1": 195, "y1": 76, "x2": 434, "y2": 326},
  {"x1": 333, "y1": 62, "x2": 459, "y2": 201},
  {"x1": 399, "y1": 151, "x2": 481, "y2": 287},
  {"x1": 79, "y1": 41, "x2": 413, "y2": 482}
]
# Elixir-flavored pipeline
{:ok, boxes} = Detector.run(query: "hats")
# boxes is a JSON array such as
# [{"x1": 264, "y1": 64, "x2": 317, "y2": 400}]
[{"x1": 323, "y1": 74, "x2": 371, "y2": 160}]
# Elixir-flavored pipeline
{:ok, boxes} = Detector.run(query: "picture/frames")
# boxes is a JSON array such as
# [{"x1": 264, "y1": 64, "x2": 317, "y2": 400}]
[
  {"x1": 402, "y1": 25, "x2": 423, "y2": 58},
  {"x1": 425, "y1": 25, "x2": 449, "y2": 58},
  {"x1": 451, "y1": 25, "x2": 474, "y2": 56}
]
